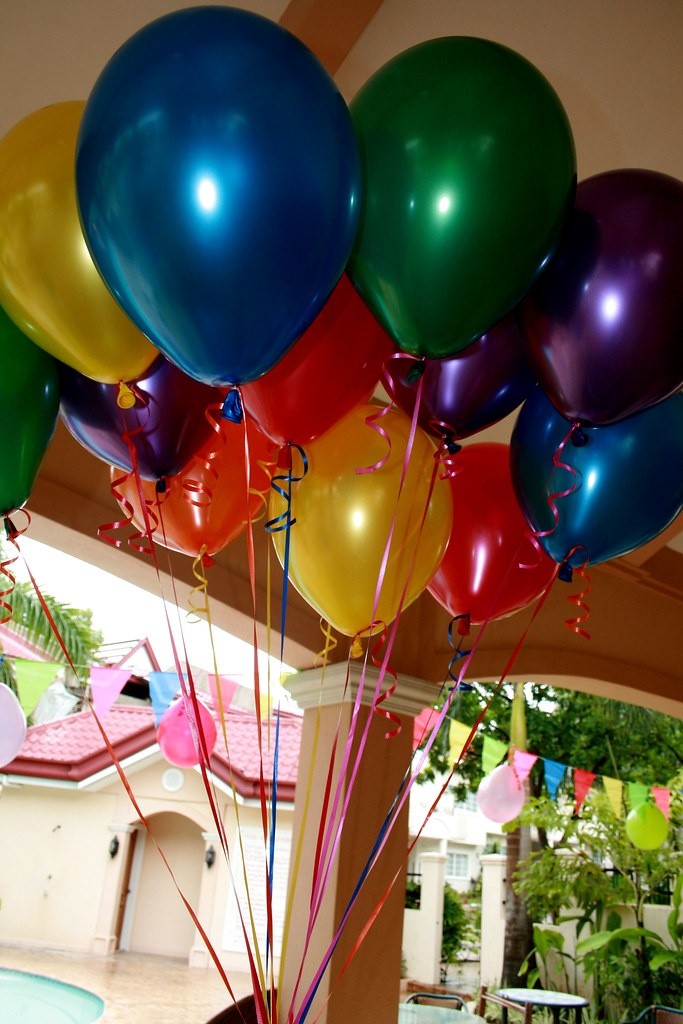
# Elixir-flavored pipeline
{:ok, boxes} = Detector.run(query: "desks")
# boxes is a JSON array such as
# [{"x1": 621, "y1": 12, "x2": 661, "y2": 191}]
[{"x1": 496, "y1": 988, "x2": 589, "y2": 1024}]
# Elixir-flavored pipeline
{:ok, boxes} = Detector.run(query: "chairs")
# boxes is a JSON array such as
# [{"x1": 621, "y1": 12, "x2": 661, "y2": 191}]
[
  {"x1": 479, "y1": 983, "x2": 532, "y2": 1024},
  {"x1": 404, "y1": 992, "x2": 469, "y2": 1014}
]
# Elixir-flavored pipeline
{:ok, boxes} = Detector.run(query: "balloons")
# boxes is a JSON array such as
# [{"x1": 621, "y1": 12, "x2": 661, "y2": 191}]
[
  {"x1": 477, "y1": 763, "x2": 526, "y2": 823},
  {"x1": 626, "y1": 799, "x2": 669, "y2": 851},
  {"x1": 1, "y1": 8, "x2": 681, "y2": 664},
  {"x1": 0, "y1": 682, "x2": 26, "y2": 774},
  {"x1": 155, "y1": 696, "x2": 219, "y2": 769}
]
